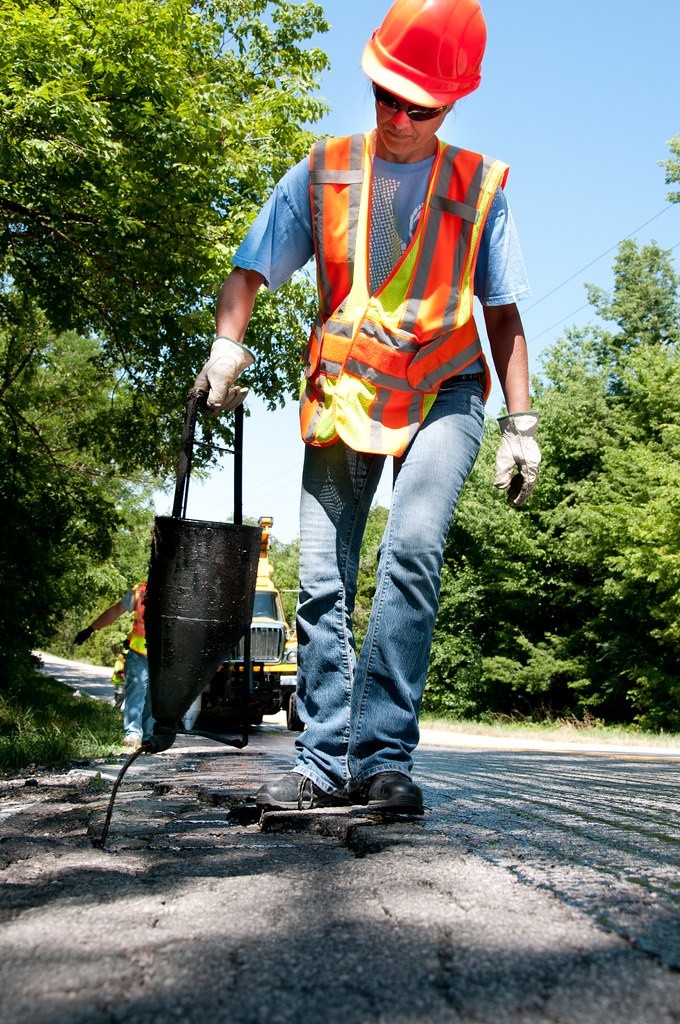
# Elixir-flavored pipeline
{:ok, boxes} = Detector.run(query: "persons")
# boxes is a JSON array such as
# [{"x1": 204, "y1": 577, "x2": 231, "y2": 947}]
[
  {"x1": 74, "y1": 581, "x2": 155, "y2": 745},
  {"x1": 191, "y1": 0, "x2": 542, "y2": 815},
  {"x1": 111, "y1": 654, "x2": 126, "y2": 710}
]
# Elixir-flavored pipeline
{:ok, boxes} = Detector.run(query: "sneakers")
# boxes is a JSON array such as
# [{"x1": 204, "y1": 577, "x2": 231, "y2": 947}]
[{"x1": 123, "y1": 734, "x2": 141, "y2": 746}]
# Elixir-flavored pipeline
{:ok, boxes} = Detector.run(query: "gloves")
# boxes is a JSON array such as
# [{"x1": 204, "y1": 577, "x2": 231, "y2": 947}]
[
  {"x1": 492, "y1": 411, "x2": 542, "y2": 504},
  {"x1": 74, "y1": 626, "x2": 95, "y2": 645},
  {"x1": 191, "y1": 336, "x2": 256, "y2": 417}
]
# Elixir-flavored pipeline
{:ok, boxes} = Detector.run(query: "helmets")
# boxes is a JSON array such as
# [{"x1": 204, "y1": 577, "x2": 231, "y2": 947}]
[{"x1": 360, "y1": 0, "x2": 486, "y2": 109}]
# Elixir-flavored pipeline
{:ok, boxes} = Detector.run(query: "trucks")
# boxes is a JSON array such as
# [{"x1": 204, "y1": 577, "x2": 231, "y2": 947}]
[{"x1": 213, "y1": 517, "x2": 306, "y2": 732}]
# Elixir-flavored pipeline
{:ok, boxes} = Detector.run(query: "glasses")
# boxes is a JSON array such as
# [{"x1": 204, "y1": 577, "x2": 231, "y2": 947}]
[{"x1": 372, "y1": 82, "x2": 450, "y2": 122}]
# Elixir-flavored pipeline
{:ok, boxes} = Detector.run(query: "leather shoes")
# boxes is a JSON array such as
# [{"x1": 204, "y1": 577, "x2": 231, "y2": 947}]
[{"x1": 256, "y1": 771, "x2": 424, "y2": 816}]
